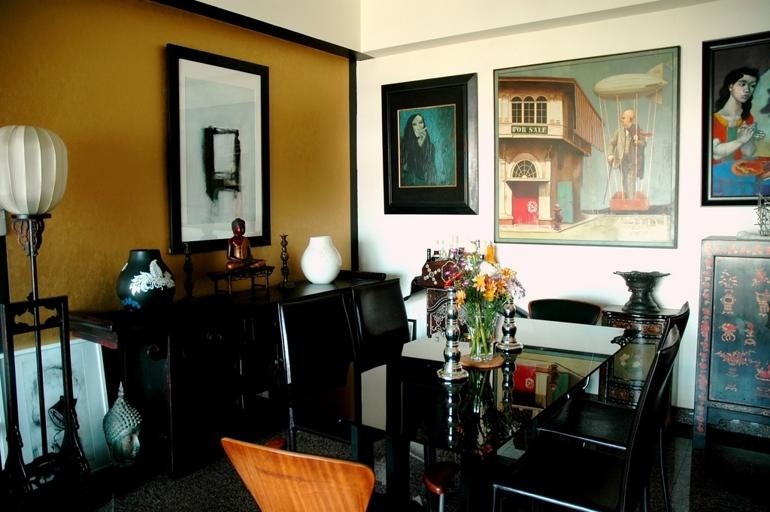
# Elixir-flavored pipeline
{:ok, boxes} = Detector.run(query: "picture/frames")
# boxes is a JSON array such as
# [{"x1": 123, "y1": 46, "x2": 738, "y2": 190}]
[
  {"x1": 699, "y1": 29, "x2": 770, "y2": 208},
  {"x1": 376, "y1": 72, "x2": 479, "y2": 215},
  {"x1": 1, "y1": 337, "x2": 114, "y2": 490},
  {"x1": 164, "y1": 41, "x2": 271, "y2": 253},
  {"x1": 493, "y1": 45, "x2": 679, "y2": 250}
]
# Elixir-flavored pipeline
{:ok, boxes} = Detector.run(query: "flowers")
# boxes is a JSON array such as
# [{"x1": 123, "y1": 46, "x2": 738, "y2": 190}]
[{"x1": 449, "y1": 244, "x2": 525, "y2": 361}]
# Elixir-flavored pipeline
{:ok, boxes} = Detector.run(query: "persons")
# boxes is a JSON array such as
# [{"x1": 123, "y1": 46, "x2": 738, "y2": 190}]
[
  {"x1": 400, "y1": 113, "x2": 438, "y2": 188},
  {"x1": 714, "y1": 67, "x2": 766, "y2": 164},
  {"x1": 606, "y1": 109, "x2": 647, "y2": 202},
  {"x1": 223, "y1": 218, "x2": 266, "y2": 272},
  {"x1": 101, "y1": 382, "x2": 144, "y2": 461}
]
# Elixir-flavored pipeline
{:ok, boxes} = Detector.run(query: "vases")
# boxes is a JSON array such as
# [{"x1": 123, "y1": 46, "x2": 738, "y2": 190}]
[
  {"x1": 301, "y1": 235, "x2": 343, "y2": 284},
  {"x1": 114, "y1": 248, "x2": 177, "y2": 314}
]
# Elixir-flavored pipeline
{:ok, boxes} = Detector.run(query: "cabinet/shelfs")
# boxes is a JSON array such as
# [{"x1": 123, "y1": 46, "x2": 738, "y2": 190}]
[
  {"x1": 690, "y1": 235, "x2": 769, "y2": 451},
  {"x1": 65, "y1": 269, "x2": 390, "y2": 480}
]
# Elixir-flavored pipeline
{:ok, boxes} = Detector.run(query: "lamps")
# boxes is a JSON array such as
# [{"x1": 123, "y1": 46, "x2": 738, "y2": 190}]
[{"x1": 0, "y1": 120, "x2": 71, "y2": 488}]
